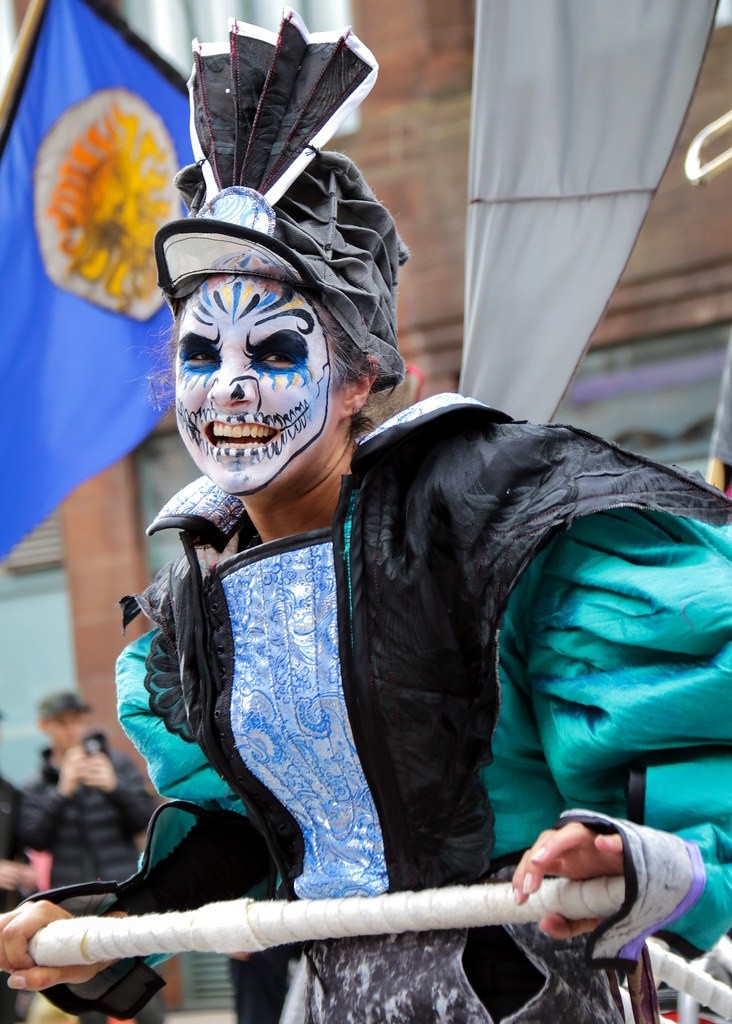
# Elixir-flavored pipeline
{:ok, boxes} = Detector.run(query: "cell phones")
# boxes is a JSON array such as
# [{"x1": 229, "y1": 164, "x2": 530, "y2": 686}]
[{"x1": 78, "y1": 732, "x2": 109, "y2": 755}]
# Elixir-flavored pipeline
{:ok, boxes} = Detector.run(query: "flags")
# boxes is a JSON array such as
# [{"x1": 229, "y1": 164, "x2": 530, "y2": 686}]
[{"x1": 0, "y1": 0, "x2": 211, "y2": 564}]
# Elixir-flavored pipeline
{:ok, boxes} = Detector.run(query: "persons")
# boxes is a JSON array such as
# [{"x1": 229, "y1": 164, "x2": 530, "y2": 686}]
[
  {"x1": 0, "y1": 5, "x2": 732, "y2": 1024},
  {"x1": 0, "y1": 695, "x2": 156, "y2": 1024}
]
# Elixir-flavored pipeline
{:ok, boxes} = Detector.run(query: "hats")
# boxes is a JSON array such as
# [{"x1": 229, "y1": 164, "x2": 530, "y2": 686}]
[
  {"x1": 35, "y1": 692, "x2": 92, "y2": 719},
  {"x1": 155, "y1": 5, "x2": 412, "y2": 394}
]
